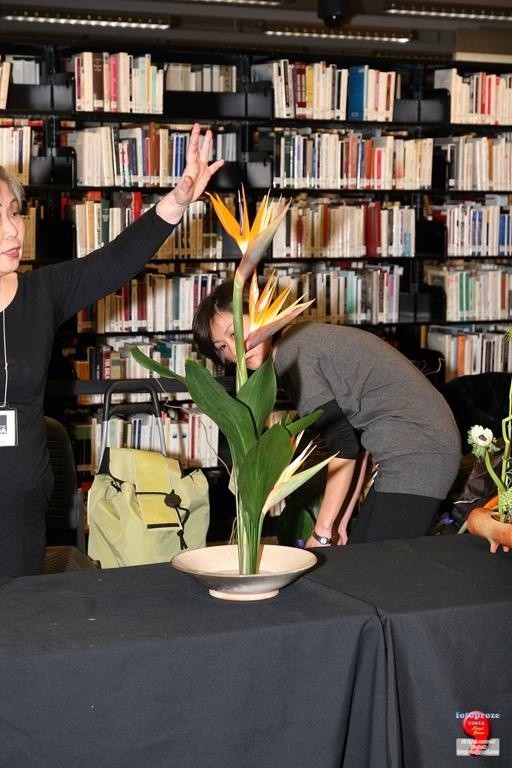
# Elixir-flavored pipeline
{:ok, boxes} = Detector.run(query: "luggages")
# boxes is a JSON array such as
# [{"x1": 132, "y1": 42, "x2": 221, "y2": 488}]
[{"x1": 84, "y1": 381, "x2": 211, "y2": 570}]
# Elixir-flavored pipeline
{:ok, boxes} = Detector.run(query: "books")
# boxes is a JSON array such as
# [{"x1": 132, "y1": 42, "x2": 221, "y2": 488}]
[{"x1": 1, "y1": 42, "x2": 512, "y2": 471}]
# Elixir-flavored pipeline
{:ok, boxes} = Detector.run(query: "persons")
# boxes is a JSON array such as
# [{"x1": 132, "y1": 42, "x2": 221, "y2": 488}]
[
  {"x1": 2, "y1": 121, "x2": 225, "y2": 580},
  {"x1": 194, "y1": 279, "x2": 464, "y2": 551}
]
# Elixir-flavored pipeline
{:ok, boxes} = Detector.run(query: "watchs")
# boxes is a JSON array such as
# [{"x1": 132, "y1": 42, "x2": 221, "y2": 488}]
[{"x1": 312, "y1": 531, "x2": 333, "y2": 545}]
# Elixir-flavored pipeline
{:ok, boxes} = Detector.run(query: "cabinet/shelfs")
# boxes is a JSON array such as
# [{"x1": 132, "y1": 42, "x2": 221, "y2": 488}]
[{"x1": 0, "y1": 0, "x2": 511, "y2": 542}]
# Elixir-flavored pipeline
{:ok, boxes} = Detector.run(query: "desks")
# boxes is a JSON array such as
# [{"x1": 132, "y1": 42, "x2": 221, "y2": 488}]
[{"x1": 0, "y1": 534, "x2": 512, "y2": 767}]
